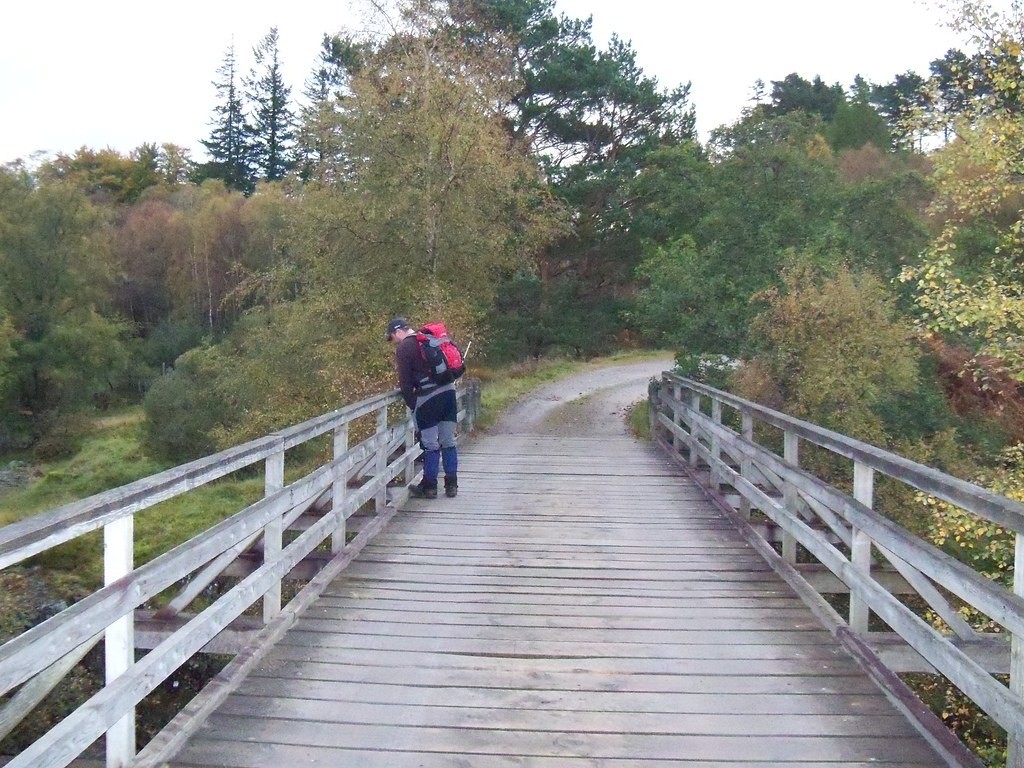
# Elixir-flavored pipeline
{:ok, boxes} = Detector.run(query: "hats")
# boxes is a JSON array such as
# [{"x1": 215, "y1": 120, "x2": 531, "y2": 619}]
[{"x1": 387, "y1": 319, "x2": 406, "y2": 341}]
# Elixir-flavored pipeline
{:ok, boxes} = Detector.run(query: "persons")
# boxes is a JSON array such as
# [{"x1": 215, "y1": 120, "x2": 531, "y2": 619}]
[{"x1": 387, "y1": 320, "x2": 459, "y2": 499}]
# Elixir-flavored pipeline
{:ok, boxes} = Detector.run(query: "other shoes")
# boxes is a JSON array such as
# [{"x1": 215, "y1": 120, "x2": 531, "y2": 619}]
[
  {"x1": 446, "y1": 485, "x2": 457, "y2": 497},
  {"x1": 408, "y1": 484, "x2": 437, "y2": 496}
]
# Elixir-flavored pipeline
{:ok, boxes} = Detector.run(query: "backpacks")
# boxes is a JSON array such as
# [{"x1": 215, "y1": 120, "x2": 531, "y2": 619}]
[{"x1": 402, "y1": 322, "x2": 465, "y2": 386}]
